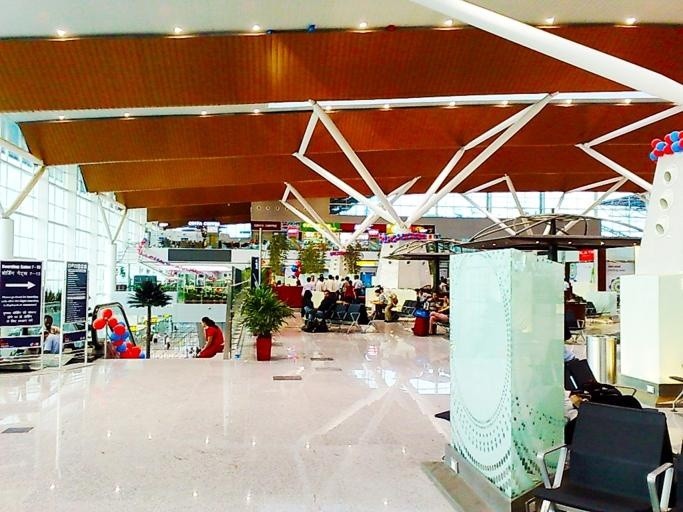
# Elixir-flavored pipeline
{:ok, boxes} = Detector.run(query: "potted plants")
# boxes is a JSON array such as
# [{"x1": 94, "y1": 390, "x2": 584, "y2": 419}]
[{"x1": 239, "y1": 285, "x2": 293, "y2": 360}]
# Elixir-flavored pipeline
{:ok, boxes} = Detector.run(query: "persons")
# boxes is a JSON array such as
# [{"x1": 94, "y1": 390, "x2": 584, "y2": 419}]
[
  {"x1": 194, "y1": 317, "x2": 225, "y2": 358},
  {"x1": 38, "y1": 314, "x2": 61, "y2": 334},
  {"x1": 300, "y1": 273, "x2": 450, "y2": 334},
  {"x1": 39, "y1": 329, "x2": 59, "y2": 355}
]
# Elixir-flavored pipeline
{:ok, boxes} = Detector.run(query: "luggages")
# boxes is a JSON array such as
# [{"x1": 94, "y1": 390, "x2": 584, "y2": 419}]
[{"x1": 411, "y1": 317, "x2": 430, "y2": 336}]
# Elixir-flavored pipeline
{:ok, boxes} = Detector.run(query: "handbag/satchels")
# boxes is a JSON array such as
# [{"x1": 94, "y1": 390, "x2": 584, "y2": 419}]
[
  {"x1": 564, "y1": 355, "x2": 597, "y2": 397},
  {"x1": 412, "y1": 307, "x2": 430, "y2": 319}
]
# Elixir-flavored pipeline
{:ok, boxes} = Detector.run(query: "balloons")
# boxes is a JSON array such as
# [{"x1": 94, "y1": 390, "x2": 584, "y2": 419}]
[
  {"x1": 289, "y1": 260, "x2": 304, "y2": 279},
  {"x1": 378, "y1": 231, "x2": 426, "y2": 244},
  {"x1": 92, "y1": 308, "x2": 145, "y2": 359},
  {"x1": 647, "y1": 130, "x2": 682, "y2": 163}
]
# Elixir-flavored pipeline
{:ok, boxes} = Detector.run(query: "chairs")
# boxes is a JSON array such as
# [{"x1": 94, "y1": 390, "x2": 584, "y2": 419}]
[
  {"x1": 525, "y1": 401, "x2": 676, "y2": 512},
  {"x1": 312, "y1": 302, "x2": 367, "y2": 333},
  {"x1": 566, "y1": 358, "x2": 642, "y2": 415},
  {"x1": 563, "y1": 302, "x2": 587, "y2": 342},
  {"x1": 392, "y1": 299, "x2": 419, "y2": 322}
]
